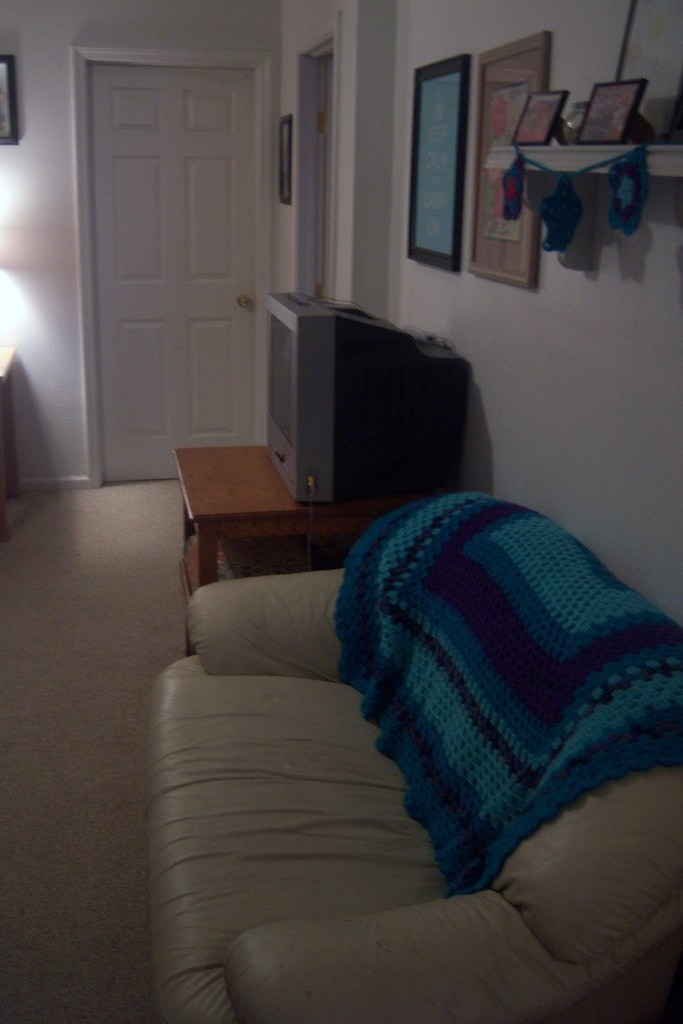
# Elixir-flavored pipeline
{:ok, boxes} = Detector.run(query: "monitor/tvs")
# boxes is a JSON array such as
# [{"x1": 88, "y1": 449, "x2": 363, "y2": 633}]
[{"x1": 263, "y1": 290, "x2": 468, "y2": 506}]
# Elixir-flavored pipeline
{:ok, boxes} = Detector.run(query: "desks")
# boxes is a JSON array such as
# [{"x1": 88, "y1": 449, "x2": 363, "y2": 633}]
[{"x1": 176, "y1": 444, "x2": 458, "y2": 669}]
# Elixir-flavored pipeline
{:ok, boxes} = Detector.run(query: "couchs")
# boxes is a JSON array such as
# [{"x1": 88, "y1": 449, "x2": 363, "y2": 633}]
[{"x1": 145, "y1": 484, "x2": 683, "y2": 1021}]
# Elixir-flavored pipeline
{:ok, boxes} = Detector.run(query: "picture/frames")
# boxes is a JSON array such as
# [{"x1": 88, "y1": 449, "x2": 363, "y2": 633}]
[
  {"x1": 470, "y1": 27, "x2": 548, "y2": 289},
  {"x1": 576, "y1": 76, "x2": 655, "y2": 145},
  {"x1": 0, "y1": 54, "x2": 20, "y2": 146},
  {"x1": 407, "y1": 51, "x2": 469, "y2": 275},
  {"x1": 513, "y1": 90, "x2": 568, "y2": 149},
  {"x1": 277, "y1": 114, "x2": 293, "y2": 205}
]
